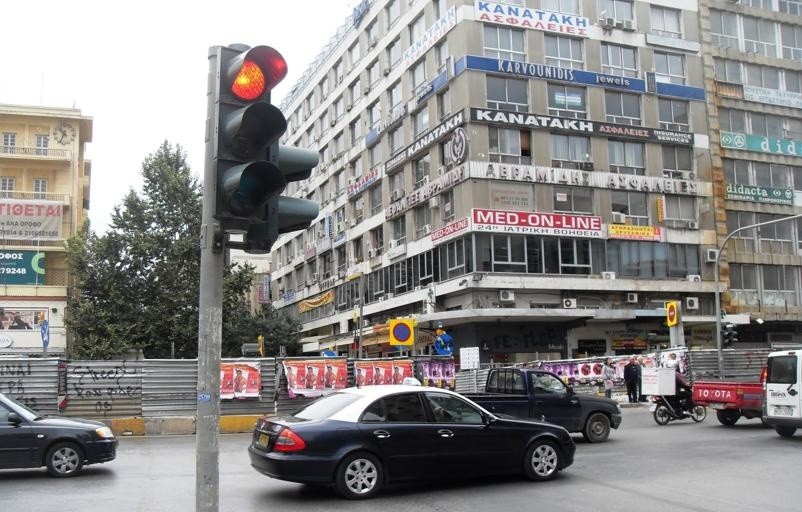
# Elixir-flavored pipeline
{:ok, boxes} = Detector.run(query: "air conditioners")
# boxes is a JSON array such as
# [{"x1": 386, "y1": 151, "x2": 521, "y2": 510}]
[
  {"x1": 279, "y1": 67, "x2": 390, "y2": 173},
  {"x1": 611, "y1": 212, "x2": 628, "y2": 225},
  {"x1": 383, "y1": 292, "x2": 394, "y2": 301},
  {"x1": 388, "y1": 240, "x2": 398, "y2": 249},
  {"x1": 428, "y1": 197, "x2": 439, "y2": 209},
  {"x1": 601, "y1": 18, "x2": 614, "y2": 32},
  {"x1": 312, "y1": 273, "x2": 319, "y2": 283},
  {"x1": 687, "y1": 221, "x2": 700, "y2": 230},
  {"x1": 360, "y1": 318, "x2": 370, "y2": 329},
  {"x1": 499, "y1": 289, "x2": 516, "y2": 303},
  {"x1": 437, "y1": 166, "x2": 448, "y2": 176},
  {"x1": 348, "y1": 177, "x2": 357, "y2": 185},
  {"x1": 601, "y1": 271, "x2": 616, "y2": 279},
  {"x1": 392, "y1": 188, "x2": 403, "y2": 200},
  {"x1": 562, "y1": 297, "x2": 578, "y2": 310},
  {"x1": 687, "y1": 273, "x2": 702, "y2": 282},
  {"x1": 415, "y1": 285, "x2": 423, "y2": 290},
  {"x1": 421, "y1": 224, "x2": 431, "y2": 236},
  {"x1": 624, "y1": 293, "x2": 639, "y2": 304},
  {"x1": 329, "y1": 191, "x2": 336, "y2": 200},
  {"x1": 685, "y1": 297, "x2": 700, "y2": 310},
  {"x1": 682, "y1": 169, "x2": 697, "y2": 183},
  {"x1": 336, "y1": 221, "x2": 344, "y2": 234},
  {"x1": 317, "y1": 230, "x2": 326, "y2": 239},
  {"x1": 348, "y1": 219, "x2": 357, "y2": 229},
  {"x1": 368, "y1": 249, "x2": 377, "y2": 260},
  {"x1": 337, "y1": 271, "x2": 346, "y2": 280},
  {"x1": 620, "y1": 20, "x2": 639, "y2": 33},
  {"x1": 370, "y1": 35, "x2": 378, "y2": 47}
]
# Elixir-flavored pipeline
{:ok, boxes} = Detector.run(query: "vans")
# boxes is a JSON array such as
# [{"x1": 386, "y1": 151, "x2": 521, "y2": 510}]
[{"x1": 764, "y1": 349, "x2": 801, "y2": 438}]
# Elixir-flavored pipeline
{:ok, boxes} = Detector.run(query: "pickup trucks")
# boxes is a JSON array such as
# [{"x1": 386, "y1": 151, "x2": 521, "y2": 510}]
[
  {"x1": 459, "y1": 367, "x2": 622, "y2": 443},
  {"x1": 692, "y1": 366, "x2": 776, "y2": 429}
]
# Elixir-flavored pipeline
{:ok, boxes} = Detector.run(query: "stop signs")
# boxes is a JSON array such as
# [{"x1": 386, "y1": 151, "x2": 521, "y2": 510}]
[{"x1": 667, "y1": 301, "x2": 678, "y2": 326}]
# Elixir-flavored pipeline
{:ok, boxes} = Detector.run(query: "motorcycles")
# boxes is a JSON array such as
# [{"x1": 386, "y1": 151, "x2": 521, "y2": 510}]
[{"x1": 641, "y1": 388, "x2": 708, "y2": 428}]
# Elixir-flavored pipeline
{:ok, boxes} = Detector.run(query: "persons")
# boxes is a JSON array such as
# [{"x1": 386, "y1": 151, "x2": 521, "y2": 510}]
[
  {"x1": 325, "y1": 364, "x2": 337, "y2": 389},
  {"x1": 355, "y1": 368, "x2": 366, "y2": 385},
  {"x1": 667, "y1": 352, "x2": 680, "y2": 372},
  {"x1": 635, "y1": 356, "x2": 649, "y2": 402},
  {"x1": 393, "y1": 366, "x2": 403, "y2": 385},
  {"x1": 305, "y1": 365, "x2": 318, "y2": 390},
  {"x1": 601, "y1": 357, "x2": 615, "y2": 398},
  {"x1": 286, "y1": 366, "x2": 297, "y2": 389},
  {"x1": 233, "y1": 370, "x2": 248, "y2": 393},
  {"x1": 623, "y1": 356, "x2": 639, "y2": 402},
  {"x1": 373, "y1": 366, "x2": 383, "y2": 385},
  {"x1": 666, "y1": 363, "x2": 692, "y2": 404}
]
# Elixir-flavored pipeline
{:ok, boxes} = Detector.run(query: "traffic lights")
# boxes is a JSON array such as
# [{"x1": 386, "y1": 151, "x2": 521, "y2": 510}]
[{"x1": 211, "y1": 41, "x2": 320, "y2": 254}]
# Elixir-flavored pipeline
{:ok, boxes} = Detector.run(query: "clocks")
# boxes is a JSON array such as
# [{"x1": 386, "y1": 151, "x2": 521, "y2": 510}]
[
  {"x1": 704, "y1": 248, "x2": 722, "y2": 266},
  {"x1": 52, "y1": 123, "x2": 79, "y2": 145}
]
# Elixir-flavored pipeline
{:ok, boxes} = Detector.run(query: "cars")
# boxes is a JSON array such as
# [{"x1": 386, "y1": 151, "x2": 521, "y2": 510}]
[
  {"x1": 0, "y1": 390, "x2": 119, "y2": 478},
  {"x1": 248, "y1": 385, "x2": 576, "y2": 500}
]
